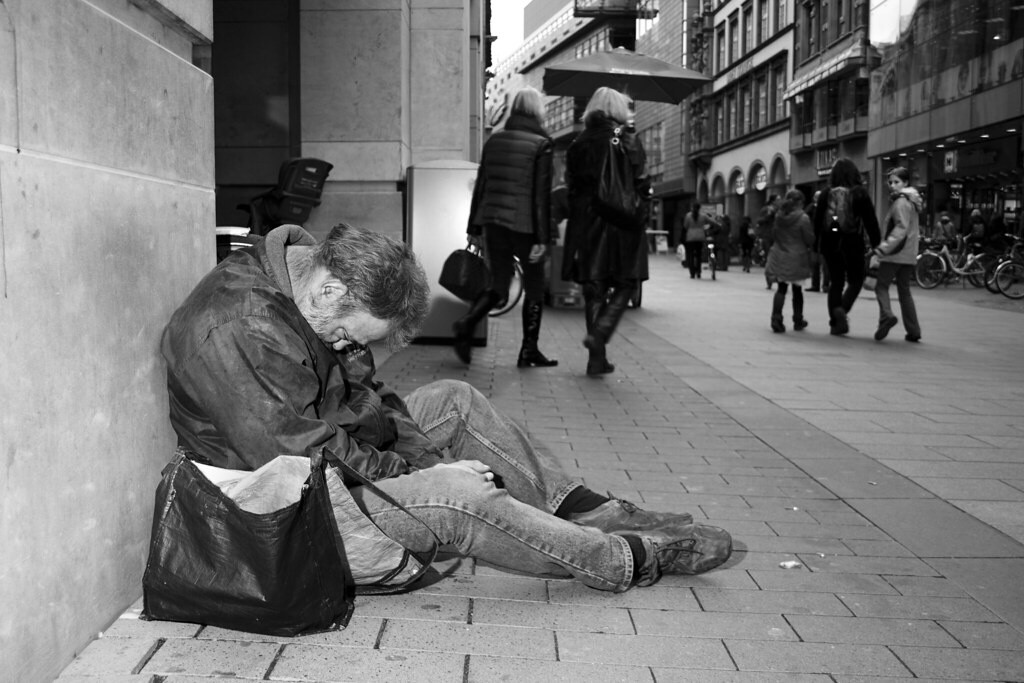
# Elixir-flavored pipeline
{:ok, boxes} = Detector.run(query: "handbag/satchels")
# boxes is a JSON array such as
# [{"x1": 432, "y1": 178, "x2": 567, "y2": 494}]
[
  {"x1": 141, "y1": 445, "x2": 443, "y2": 636},
  {"x1": 439, "y1": 239, "x2": 492, "y2": 302},
  {"x1": 748, "y1": 224, "x2": 755, "y2": 236},
  {"x1": 951, "y1": 238, "x2": 957, "y2": 249},
  {"x1": 599, "y1": 125, "x2": 652, "y2": 226},
  {"x1": 885, "y1": 217, "x2": 908, "y2": 255}
]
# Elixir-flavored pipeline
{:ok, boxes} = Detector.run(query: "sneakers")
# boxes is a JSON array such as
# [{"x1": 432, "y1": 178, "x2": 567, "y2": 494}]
[
  {"x1": 604, "y1": 524, "x2": 733, "y2": 588},
  {"x1": 566, "y1": 490, "x2": 694, "y2": 533}
]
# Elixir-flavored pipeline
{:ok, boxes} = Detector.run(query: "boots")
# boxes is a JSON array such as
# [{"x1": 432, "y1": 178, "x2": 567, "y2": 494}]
[
  {"x1": 518, "y1": 275, "x2": 558, "y2": 368},
  {"x1": 583, "y1": 297, "x2": 615, "y2": 376},
  {"x1": 452, "y1": 287, "x2": 502, "y2": 365},
  {"x1": 583, "y1": 288, "x2": 630, "y2": 357}
]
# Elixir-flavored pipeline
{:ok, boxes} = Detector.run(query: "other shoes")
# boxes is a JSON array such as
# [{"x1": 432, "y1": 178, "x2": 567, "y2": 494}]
[
  {"x1": 906, "y1": 335, "x2": 921, "y2": 341},
  {"x1": 771, "y1": 315, "x2": 785, "y2": 333},
  {"x1": 792, "y1": 315, "x2": 808, "y2": 330},
  {"x1": 823, "y1": 285, "x2": 829, "y2": 293},
  {"x1": 805, "y1": 286, "x2": 819, "y2": 292},
  {"x1": 830, "y1": 308, "x2": 849, "y2": 335},
  {"x1": 874, "y1": 315, "x2": 898, "y2": 340}
]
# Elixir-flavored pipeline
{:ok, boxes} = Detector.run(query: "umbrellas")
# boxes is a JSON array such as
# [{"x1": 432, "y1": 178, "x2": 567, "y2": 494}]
[{"x1": 541, "y1": 47, "x2": 713, "y2": 106}]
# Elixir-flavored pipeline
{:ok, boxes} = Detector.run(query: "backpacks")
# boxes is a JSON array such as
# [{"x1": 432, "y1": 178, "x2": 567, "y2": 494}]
[{"x1": 822, "y1": 184, "x2": 860, "y2": 236}]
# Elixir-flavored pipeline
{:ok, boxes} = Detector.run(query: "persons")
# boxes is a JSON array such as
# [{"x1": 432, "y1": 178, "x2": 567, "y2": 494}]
[
  {"x1": 761, "y1": 194, "x2": 780, "y2": 289},
  {"x1": 865, "y1": 166, "x2": 922, "y2": 342},
  {"x1": 739, "y1": 216, "x2": 755, "y2": 273},
  {"x1": 160, "y1": 222, "x2": 733, "y2": 595},
  {"x1": 805, "y1": 191, "x2": 828, "y2": 293},
  {"x1": 916, "y1": 206, "x2": 1006, "y2": 255},
  {"x1": 812, "y1": 158, "x2": 882, "y2": 335},
  {"x1": 561, "y1": 86, "x2": 650, "y2": 376},
  {"x1": 717, "y1": 215, "x2": 731, "y2": 271},
  {"x1": 452, "y1": 87, "x2": 559, "y2": 367},
  {"x1": 684, "y1": 203, "x2": 722, "y2": 279},
  {"x1": 764, "y1": 189, "x2": 809, "y2": 332}
]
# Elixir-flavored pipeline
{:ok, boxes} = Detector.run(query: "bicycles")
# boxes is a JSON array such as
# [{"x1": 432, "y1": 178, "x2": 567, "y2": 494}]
[
  {"x1": 706, "y1": 226, "x2": 716, "y2": 282},
  {"x1": 911, "y1": 229, "x2": 1024, "y2": 300},
  {"x1": 486, "y1": 255, "x2": 525, "y2": 317}
]
